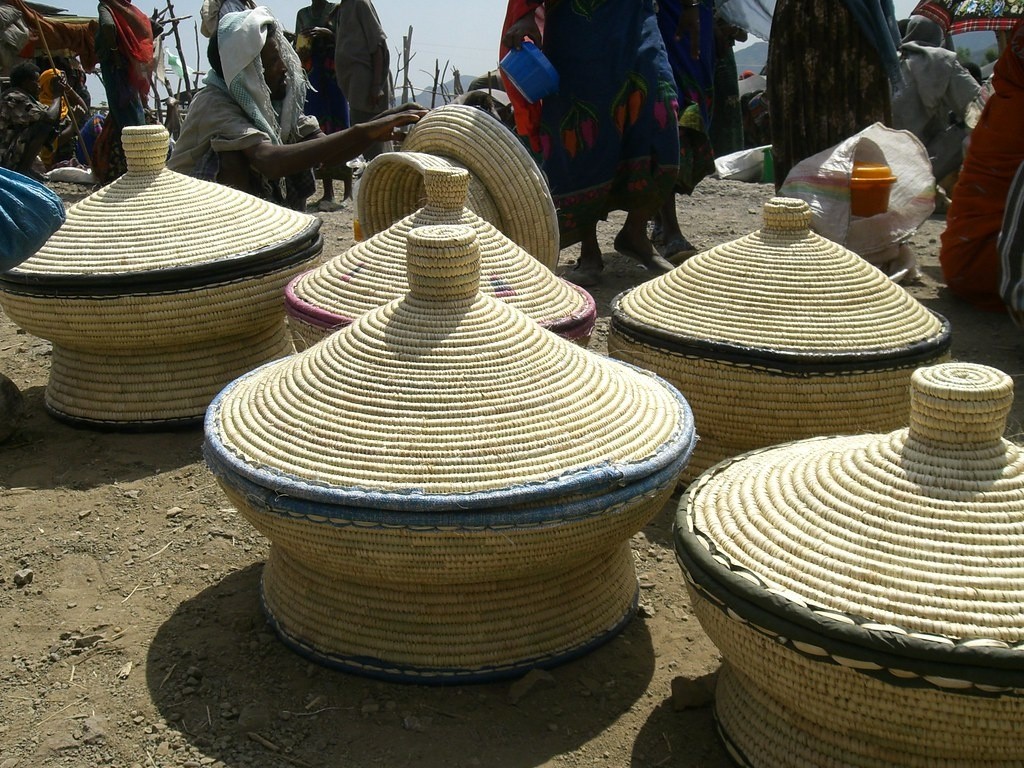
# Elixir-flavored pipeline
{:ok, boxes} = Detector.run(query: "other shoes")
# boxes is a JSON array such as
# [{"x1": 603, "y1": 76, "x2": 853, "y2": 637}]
[
  {"x1": 573, "y1": 254, "x2": 604, "y2": 287},
  {"x1": 612, "y1": 230, "x2": 675, "y2": 273},
  {"x1": 649, "y1": 230, "x2": 697, "y2": 261}
]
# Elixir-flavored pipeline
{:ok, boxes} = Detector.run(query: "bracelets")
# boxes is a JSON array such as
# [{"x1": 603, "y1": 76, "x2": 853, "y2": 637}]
[
  {"x1": 110, "y1": 47, "x2": 118, "y2": 51},
  {"x1": 66, "y1": 87, "x2": 72, "y2": 93}
]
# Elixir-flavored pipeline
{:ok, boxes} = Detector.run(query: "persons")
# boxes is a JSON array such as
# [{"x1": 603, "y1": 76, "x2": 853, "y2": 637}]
[
  {"x1": 167, "y1": 6, "x2": 431, "y2": 211},
  {"x1": 650, "y1": 1, "x2": 1024, "y2": 303},
  {"x1": 200, "y1": 0, "x2": 296, "y2": 44},
  {"x1": 95, "y1": 0, "x2": 164, "y2": 182},
  {"x1": 499, "y1": 0, "x2": 681, "y2": 284},
  {"x1": 282, "y1": 0, "x2": 394, "y2": 210},
  {"x1": 0, "y1": 61, "x2": 88, "y2": 181}
]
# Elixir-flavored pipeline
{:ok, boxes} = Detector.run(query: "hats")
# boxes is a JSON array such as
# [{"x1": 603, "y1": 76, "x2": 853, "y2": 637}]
[{"x1": 39, "y1": 68, "x2": 61, "y2": 86}]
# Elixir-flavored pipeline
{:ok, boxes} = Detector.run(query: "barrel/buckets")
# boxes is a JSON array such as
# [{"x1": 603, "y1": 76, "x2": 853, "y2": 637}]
[{"x1": 849, "y1": 162, "x2": 897, "y2": 218}]
